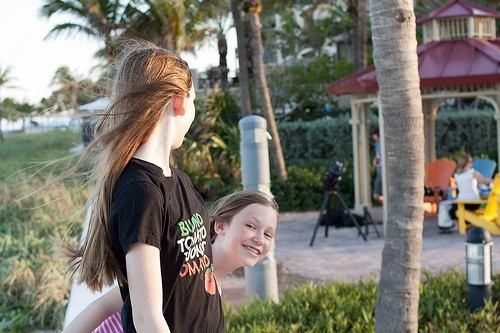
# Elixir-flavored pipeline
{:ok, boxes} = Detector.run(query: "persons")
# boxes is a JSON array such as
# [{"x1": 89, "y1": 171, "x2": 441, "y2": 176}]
[
  {"x1": 370, "y1": 128, "x2": 382, "y2": 198},
  {"x1": 8, "y1": 38, "x2": 226, "y2": 333},
  {"x1": 439, "y1": 154, "x2": 492, "y2": 228},
  {"x1": 61, "y1": 189, "x2": 279, "y2": 333}
]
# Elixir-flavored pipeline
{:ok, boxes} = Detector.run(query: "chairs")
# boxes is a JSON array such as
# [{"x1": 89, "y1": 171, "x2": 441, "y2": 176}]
[
  {"x1": 423, "y1": 157, "x2": 456, "y2": 219},
  {"x1": 453, "y1": 174, "x2": 500, "y2": 236},
  {"x1": 447, "y1": 158, "x2": 496, "y2": 200}
]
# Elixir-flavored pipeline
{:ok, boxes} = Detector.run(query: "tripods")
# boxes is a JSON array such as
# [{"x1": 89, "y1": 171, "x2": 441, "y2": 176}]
[{"x1": 308, "y1": 188, "x2": 368, "y2": 247}]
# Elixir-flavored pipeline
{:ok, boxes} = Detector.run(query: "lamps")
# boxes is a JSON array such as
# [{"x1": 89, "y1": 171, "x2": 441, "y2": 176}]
[{"x1": 463, "y1": 228, "x2": 493, "y2": 313}]
